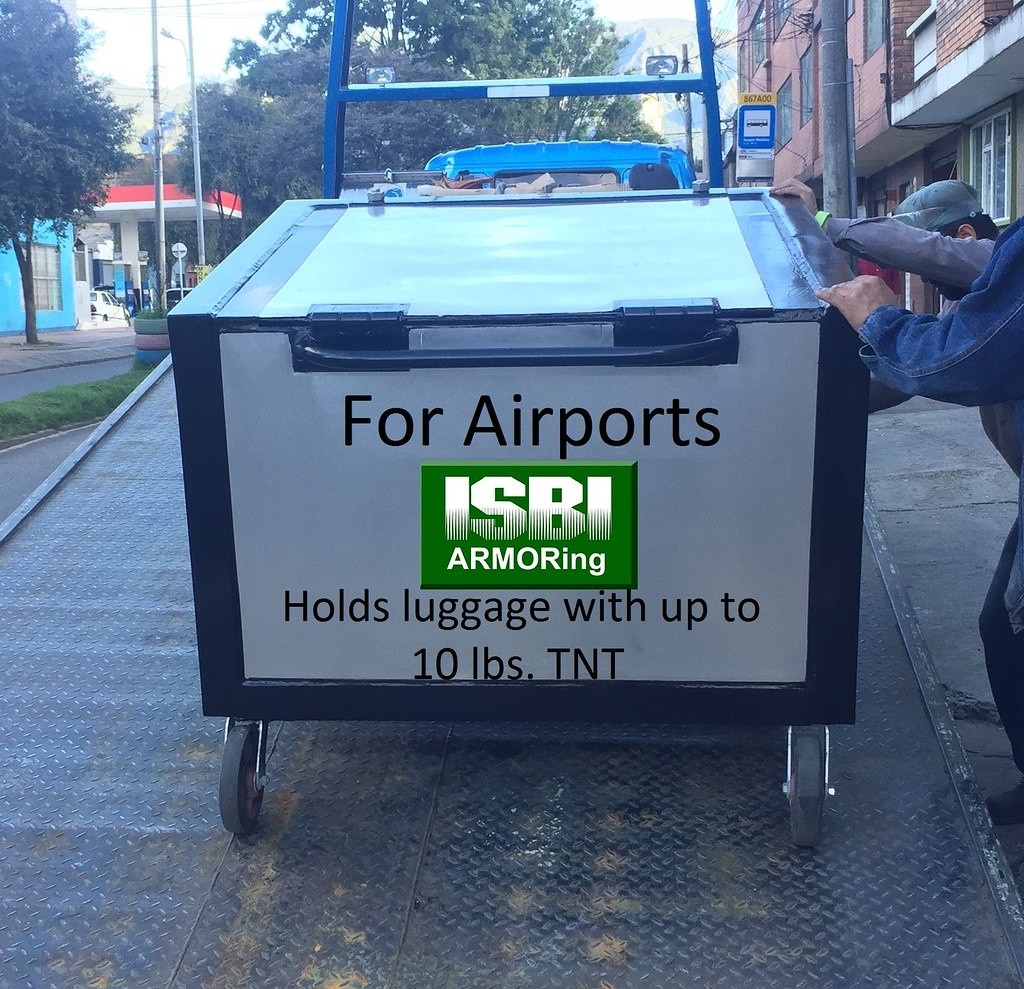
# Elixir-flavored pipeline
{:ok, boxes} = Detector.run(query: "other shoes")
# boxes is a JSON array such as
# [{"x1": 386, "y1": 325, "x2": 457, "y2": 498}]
[{"x1": 985, "y1": 780, "x2": 1024, "y2": 825}]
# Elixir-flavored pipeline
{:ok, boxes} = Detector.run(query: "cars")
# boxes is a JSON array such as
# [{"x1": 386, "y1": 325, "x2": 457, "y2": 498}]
[
  {"x1": 90, "y1": 291, "x2": 131, "y2": 323},
  {"x1": 165, "y1": 288, "x2": 193, "y2": 307},
  {"x1": 94, "y1": 285, "x2": 139, "y2": 315}
]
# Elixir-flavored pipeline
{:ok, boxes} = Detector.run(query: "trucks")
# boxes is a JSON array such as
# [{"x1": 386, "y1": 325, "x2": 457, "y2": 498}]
[{"x1": 3, "y1": 144, "x2": 1024, "y2": 987}]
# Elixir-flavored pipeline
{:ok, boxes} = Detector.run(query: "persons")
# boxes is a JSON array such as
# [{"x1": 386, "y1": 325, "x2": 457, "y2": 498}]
[{"x1": 766, "y1": 176, "x2": 1024, "y2": 824}]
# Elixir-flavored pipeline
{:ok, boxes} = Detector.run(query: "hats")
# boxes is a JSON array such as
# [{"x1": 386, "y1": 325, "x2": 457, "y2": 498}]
[{"x1": 893, "y1": 179, "x2": 984, "y2": 233}]
